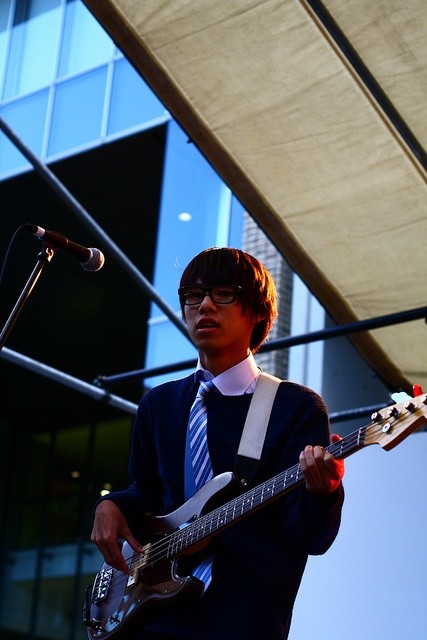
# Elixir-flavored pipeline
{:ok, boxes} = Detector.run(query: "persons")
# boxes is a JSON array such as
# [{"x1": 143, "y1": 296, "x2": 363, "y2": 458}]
[{"x1": 89, "y1": 246, "x2": 347, "y2": 640}]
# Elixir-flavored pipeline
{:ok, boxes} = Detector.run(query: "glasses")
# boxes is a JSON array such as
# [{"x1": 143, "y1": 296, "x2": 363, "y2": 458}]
[{"x1": 177, "y1": 283, "x2": 245, "y2": 304}]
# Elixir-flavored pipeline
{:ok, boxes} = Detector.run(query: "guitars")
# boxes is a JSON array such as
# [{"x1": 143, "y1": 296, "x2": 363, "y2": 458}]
[{"x1": 80, "y1": 393, "x2": 426, "y2": 639}]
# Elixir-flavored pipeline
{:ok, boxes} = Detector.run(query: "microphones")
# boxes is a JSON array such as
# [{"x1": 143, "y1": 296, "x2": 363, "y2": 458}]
[{"x1": 29, "y1": 223, "x2": 105, "y2": 272}]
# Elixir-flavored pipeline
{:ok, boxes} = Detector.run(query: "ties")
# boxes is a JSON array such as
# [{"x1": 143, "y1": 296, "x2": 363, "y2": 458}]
[{"x1": 184, "y1": 375, "x2": 216, "y2": 597}]
